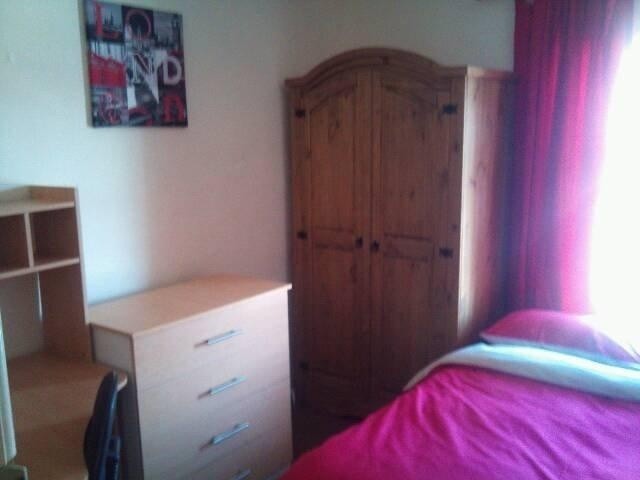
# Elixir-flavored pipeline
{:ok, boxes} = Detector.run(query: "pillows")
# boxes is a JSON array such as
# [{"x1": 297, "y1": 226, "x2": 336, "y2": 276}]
[{"x1": 478, "y1": 308, "x2": 640, "y2": 371}]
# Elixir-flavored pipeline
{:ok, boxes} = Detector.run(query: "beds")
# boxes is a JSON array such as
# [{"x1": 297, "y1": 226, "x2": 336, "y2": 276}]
[{"x1": 277, "y1": 306, "x2": 640, "y2": 480}]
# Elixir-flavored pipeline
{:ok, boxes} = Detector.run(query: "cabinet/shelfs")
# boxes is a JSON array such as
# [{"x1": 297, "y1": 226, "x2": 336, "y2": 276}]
[
  {"x1": 89, "y1": 273, "x2": 294, "y2": 480},
  {"x1": 285, "y1": 46, "x2": 512, "y2": 419},
  {"x1": 1, "y1": 184, "x2": 84, "y2": 279}
]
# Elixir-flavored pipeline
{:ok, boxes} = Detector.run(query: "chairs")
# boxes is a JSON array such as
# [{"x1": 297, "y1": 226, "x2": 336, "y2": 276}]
[{"x1": 85, "y1": 369, "x2": 121, "y2": 479}]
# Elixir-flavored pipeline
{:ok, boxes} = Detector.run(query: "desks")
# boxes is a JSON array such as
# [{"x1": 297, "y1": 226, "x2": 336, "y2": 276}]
[{"x1": 0, "y1": 352, "x2": 129, "y2": 480}]
